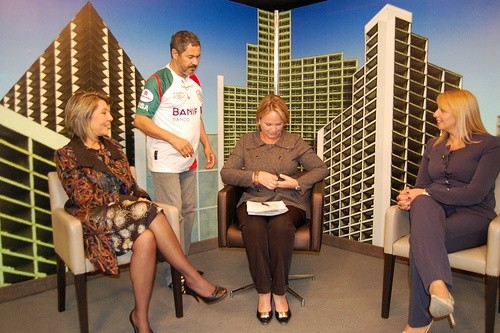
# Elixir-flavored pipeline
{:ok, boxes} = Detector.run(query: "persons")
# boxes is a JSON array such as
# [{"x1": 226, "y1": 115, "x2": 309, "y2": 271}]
[
  {"x1": 54, "y1": 91, "x2": 227, "y2": 333},
  {"x1": 396, "y1": 89, "x2": 500, "y2": 333},
  {"x1": 132, "y1": 30, "x2": 216, "y2": 294},
  {"x1": 220, "y1": 94, "x2": 329, "y2": 326}
]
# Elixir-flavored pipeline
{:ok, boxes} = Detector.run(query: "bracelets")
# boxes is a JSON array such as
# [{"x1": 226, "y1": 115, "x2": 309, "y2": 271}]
[
  {"x1": 253, "y1": 170, "x2": 260, "y2": 186},
  {"x1": 422, "y1": 188, "x2": 431, "y2": 196}
]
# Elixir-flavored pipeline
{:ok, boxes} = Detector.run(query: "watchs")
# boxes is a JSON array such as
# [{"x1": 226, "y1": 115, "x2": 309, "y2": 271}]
[{"x1": 295, "y1": 185, "x2": 301, "y2": 190}]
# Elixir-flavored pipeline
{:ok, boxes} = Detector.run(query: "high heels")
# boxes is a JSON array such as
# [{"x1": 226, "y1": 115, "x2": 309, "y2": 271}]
[
  {"x1": 401, "y1": 318, "x2": 434, "y2": 333},
  {"x1": 129, "y1": 310, "x2": 153, "y2": 333},
  {"x1": 428, "y1": 292, "x2": 456, "y2": 328},
  {"x1": 184, "y1": 281, "x2": 227, "y2": 305}
]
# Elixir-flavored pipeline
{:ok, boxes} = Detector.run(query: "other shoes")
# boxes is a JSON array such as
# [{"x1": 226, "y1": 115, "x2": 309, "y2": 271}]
[{"x1": 170, "y1": 270, "x2": 204, "y2": 294}]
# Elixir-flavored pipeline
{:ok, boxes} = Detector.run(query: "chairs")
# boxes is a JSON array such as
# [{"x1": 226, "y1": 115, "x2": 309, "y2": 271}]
[
  {"x1": 381, "y1": 174, "x2": 500, "y2": 333},
  {"x1": 217, "y1": 177, "x2": 325, "y2": 307},
  {"x1": 48, "y1": 165, "x2": 184, "y2": 333}
]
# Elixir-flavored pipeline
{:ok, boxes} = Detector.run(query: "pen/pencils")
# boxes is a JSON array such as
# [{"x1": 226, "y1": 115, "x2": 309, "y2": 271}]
[{"x1": 261, "y1": 202, "x2": 270, "y2": 208}]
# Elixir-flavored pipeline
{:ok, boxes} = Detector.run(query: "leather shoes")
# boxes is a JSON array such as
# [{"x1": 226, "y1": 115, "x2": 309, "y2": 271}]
[
  {"x1": 256, "y1": 292, "x2": 272, "y2": 325},
  {"x1": 275, "y1": 296, "x2": 291, "y2": 325}
]
